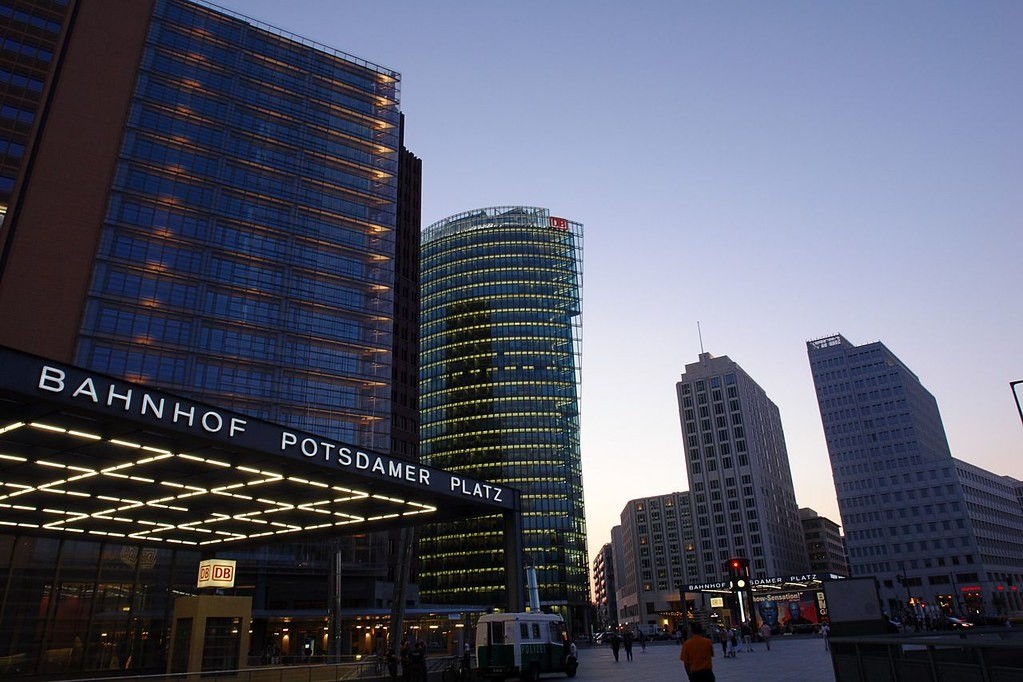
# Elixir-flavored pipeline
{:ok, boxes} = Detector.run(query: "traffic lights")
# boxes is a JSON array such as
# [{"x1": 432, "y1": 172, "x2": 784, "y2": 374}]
[{"x1": 726, "y1": 558, "x2": 749, "y2": 569}]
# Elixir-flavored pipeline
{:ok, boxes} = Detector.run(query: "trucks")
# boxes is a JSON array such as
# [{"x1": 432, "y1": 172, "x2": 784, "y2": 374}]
[
  {"x1": 475, "y1": 613, "x2": 579, "y2": 682},
  {"x1": 638, "y1": 627, "x2": 672, "y2": 637}
]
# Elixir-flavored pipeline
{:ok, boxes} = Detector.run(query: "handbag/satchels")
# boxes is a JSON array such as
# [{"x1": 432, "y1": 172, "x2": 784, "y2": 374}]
[
  {"x1": 731, "y1": 638, "x2": 737, "y2": 646},
  {"x1": 825, "y1": 629, "x2": 830, "y2": 637}
]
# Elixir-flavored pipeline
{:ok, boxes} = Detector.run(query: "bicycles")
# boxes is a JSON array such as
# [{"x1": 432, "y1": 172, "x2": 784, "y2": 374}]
[{"x1": 443, "y1": 655, "x2": 473, "y2": 681}]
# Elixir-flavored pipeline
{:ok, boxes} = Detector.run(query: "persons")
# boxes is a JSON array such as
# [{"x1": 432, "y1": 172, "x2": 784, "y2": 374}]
[
  {"x1": 758, "y1": 601, "x2": 782, "y2": 631},
  {"x1": 676, "y1": 626, "x2": 687, "y2": 645},
  {"x1": 893, "y1": 614, "x2": 934, "y2": 632},
  {"x1": 784, "y1": 602, "x2": 814, "y2": 628},
  {"x1": 680, "y1": 623, "x2": 715, "y2": 682},
  {"x1": 640, "y1": 633, "x2": 646, "y2": 652},
  {"x1": 719, "y1": 621, "x2": 771, "y2": 658},
  {"x1": 70, "y1": 636, "x2": 83, "y2": 670},
  {"x1": 821, "y1": 622, "x2": 830, "y2": 650},
  {"x1": 612, "y1": 635, "x2": 620, "y2": 662},
  {"x1": 388, "y1": 640, "x2": 427, "y2": 682},
  {"x1": 624, "y1": 636, "x2": 632, "y2": 661},
  {"x1": 998, "y1": 617, "x2": 1016, "y2": 640}
]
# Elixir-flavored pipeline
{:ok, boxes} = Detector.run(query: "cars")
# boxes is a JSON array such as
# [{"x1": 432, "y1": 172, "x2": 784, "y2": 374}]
[
  {"x1": 946, "y1": 617, "x2": 974, "y2": 630},
  {"x1": 575, "y1": 631, "x2": 624, "y2": 644}
]
